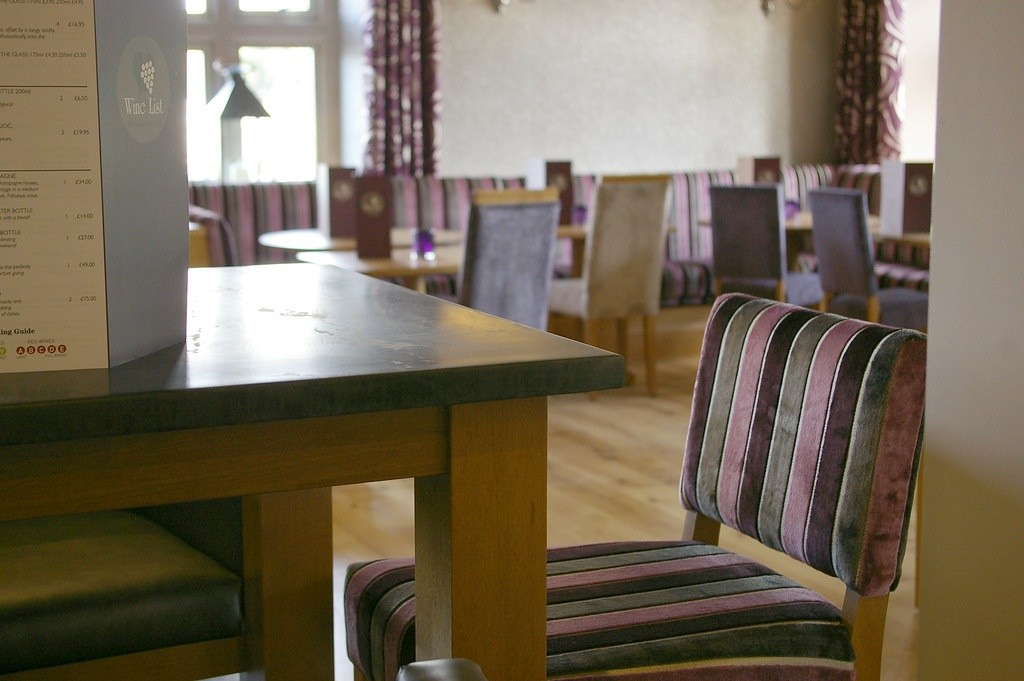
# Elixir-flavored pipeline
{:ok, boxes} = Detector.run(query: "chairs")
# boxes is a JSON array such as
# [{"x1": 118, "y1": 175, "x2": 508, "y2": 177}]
[
  {"x1": 553, "y1": 174, "x2": 674, "y2": 398},
  {"x1": 456, "y1": 188, "x2": 562, "y2": 331},
  {"x1": 707, "y1": 182, "x2": 826, "y2": 314},
  {"x1": 806, "y1": 187, "x2": 928, "y2": 333},
  {"x1": 345, "y1": 292, "x2": 927, "y2": 681},
  {"x1": 0, "y1": 486, "x2": 246, "y2": 681}
]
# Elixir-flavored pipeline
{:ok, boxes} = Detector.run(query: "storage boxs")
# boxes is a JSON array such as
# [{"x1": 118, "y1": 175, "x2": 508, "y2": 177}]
[{"x1": 1, "y1": 0, "x2": 188, "y2": 372}]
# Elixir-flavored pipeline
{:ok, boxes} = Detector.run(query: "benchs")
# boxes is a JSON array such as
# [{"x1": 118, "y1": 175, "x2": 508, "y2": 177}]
[
  {"x1": 569, "y1": 163, "x2": 836, "y2": 309},
  {"x1": 188, "y1": 178, "x2": 562, "y2": 304},
  {"x1": 836, "y1": 163, "x2": 930, "y2": 293}
]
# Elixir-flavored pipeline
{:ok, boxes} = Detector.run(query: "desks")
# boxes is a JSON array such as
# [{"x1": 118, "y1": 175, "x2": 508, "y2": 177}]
[
  {"x1": 697, "y1": 208, "x2": 931, "y2": 271},
  {"x1": 254, "y1": 227, "x2": 465, "y2": 294},
  {"x1": 0, "y1": 264, "x2": 625, "y2": 680}
]
